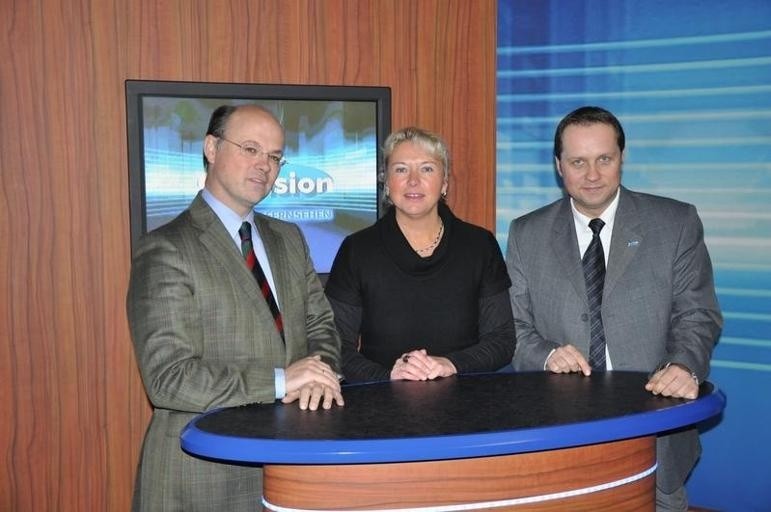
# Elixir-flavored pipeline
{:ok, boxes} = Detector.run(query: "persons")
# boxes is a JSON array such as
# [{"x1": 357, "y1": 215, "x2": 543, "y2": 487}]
[
  {"x1": 126, "y1": 104, "x2": 344, "y2": 512},
  {"x1": 503, "y1": 105, "x2": 724, "y2": 511},
  {"x1": 324, "y1": 125, "x2": 517, "y2": 385}
]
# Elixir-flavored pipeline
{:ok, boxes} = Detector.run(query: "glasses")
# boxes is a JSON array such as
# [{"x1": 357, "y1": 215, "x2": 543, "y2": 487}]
[{"x1": 219, "y1": 138, "x2": 286, "y2": 166}]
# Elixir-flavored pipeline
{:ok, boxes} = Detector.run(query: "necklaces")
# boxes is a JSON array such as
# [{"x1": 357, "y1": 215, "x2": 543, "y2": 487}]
[{"x1": 415, "y1": 222, "x2": 444, "y2": 255}]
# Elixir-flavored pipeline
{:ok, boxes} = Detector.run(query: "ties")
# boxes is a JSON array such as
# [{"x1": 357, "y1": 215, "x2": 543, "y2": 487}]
[
  {"x1": 582, "y1": 218, "x2": 606, "y2": 373},
  {"x1": 239, "y1": 222, "x2": 284, "y2": 336}
]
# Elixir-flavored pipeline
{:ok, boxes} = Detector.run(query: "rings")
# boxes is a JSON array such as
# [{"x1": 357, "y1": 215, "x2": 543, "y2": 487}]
[{"x1": 403, "y1": 355, "x2": 411, "y2": 363}]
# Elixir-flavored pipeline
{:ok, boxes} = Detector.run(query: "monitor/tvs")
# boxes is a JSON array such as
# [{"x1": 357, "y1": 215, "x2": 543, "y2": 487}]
[{"x1": 122, "y1": 78, "x2": 393, "y2": 294}]
